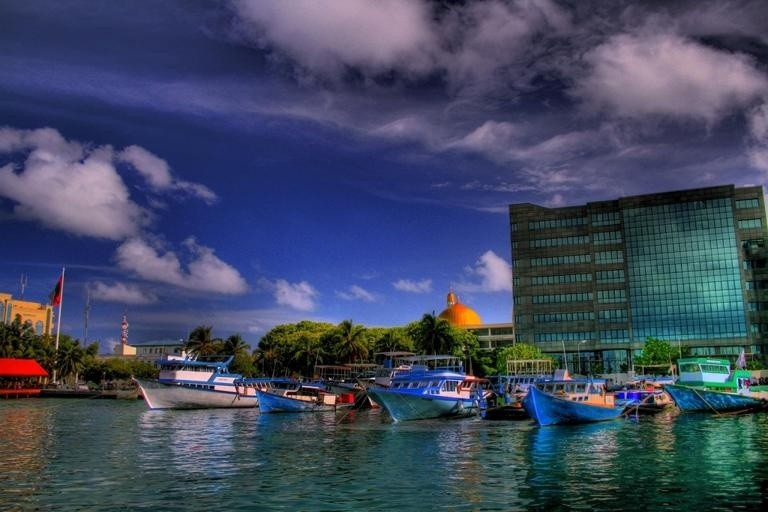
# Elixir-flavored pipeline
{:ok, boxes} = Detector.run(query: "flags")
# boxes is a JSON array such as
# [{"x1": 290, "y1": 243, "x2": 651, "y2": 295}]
[
  {"x1": 735, "y1": 349, "x2": 747, "y2": 368},
  {"x1": 47, "y1": 272, "x2": 64, "y2": 308}
]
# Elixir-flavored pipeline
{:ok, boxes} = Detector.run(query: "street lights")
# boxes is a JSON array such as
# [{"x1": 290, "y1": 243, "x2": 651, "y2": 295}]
[{"x1": 576, "y1": 340, "x2": 587, "y2": 374}]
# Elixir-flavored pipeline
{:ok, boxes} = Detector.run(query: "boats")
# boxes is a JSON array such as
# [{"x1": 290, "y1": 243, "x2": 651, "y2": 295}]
[{"x1": 131, "y1": 347, "x2": 269, "y2": 411}]
